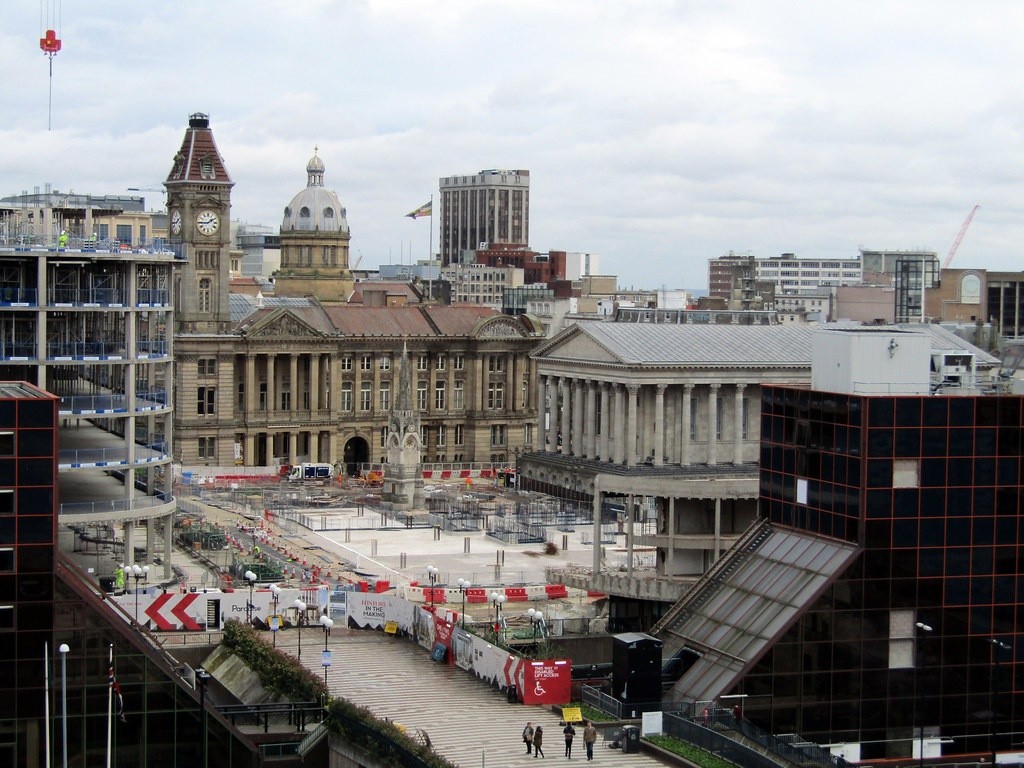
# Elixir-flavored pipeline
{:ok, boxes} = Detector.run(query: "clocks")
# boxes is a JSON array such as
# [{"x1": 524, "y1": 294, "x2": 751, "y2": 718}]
[
  {"x1": 197, "y1": 210, "x2": 220, "y2": 236},
  {"x1": 171, "y1": 210, "x2": 182, "y2": 235}
]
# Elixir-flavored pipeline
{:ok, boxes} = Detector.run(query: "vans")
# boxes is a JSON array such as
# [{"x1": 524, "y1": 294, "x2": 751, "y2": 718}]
[{"x1": 288, "y1": 463, "x2": 334, "y2": 485}]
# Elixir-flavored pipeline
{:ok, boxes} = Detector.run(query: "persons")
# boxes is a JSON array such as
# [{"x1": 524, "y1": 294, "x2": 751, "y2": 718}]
[
  {"x1": 837, "y1": 753, "x2": 846, "y2": 768},
  {"x1": 532, "y1": 726, "x2": 545, "y2": 758},
  {"x1": 115, "y1": 564, "x2": 124, "y2": 585},
  {"x1": 521, "y1": 722, "x2": 534, "y2": 754},
  {"x1": 466, "y1": 476, "x2": 472, "y2": 490},
  {"x1": 60, "y1": 231, "x2": 67, "y2": 248},
  {"x1": 583, "y1": 722, "x2": 597, "y2": 761},
  {"x1": 733, "y1": 705, "x2": 743, "y2": 731},
  {"x1": 563, "y1": 722, "x2": 576, "y2": 759},
  {"x1": 89, "y1": 232, "x2": 97, "y2": 241}
]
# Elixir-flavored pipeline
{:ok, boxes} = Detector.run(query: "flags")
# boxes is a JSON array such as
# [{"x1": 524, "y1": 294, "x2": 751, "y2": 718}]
[{"x1": 404, "y1": 201, "x2": 432, "y2": 220}]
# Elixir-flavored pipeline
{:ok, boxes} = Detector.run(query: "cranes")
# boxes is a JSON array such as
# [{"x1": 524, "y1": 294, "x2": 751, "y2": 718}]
[{"x1": 938, "y1": 204, "x2": 981, "y2": 283}]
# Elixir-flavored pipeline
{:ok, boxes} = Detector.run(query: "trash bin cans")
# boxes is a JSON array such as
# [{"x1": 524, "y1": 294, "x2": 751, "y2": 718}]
[
  {"x1": 622, "y1": 725, "x2": 640, "y2": 754},
  {"x1": 508, "y1": 685, "x2": 516, "y2": 704},
  {"x1": 99, "y1": 576, "x2": 116, "y2": 592}
]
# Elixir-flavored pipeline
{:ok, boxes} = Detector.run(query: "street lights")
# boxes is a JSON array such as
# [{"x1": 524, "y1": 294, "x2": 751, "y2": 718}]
[
  {"x1": 319, "y1": 615, "x2": 333, "y2": 686},
  {"x1": 528, "y1": 608, "x2": 543, "y2": 653},
  {"x1": 270, "y1": 584, "x2": 281, "y2": 648},
  {"x1": 245, "y1": 570, "x2": 257, "y2": 626},
  {"x1": 124, "y1": 565, "x2": 150, "y2": 621},
  {"x1": 457, "y1": 578, "x2": 470, "y2": 630},
  {"x1": 426, "y1": 565, "x2": 439, "y2": 607},
  {"x1": 294, "y1": 598, "x2": 307, "y2": 661},
  {"x1": 491, "y1": 592, "x2": 506, "y2": 647}
]
